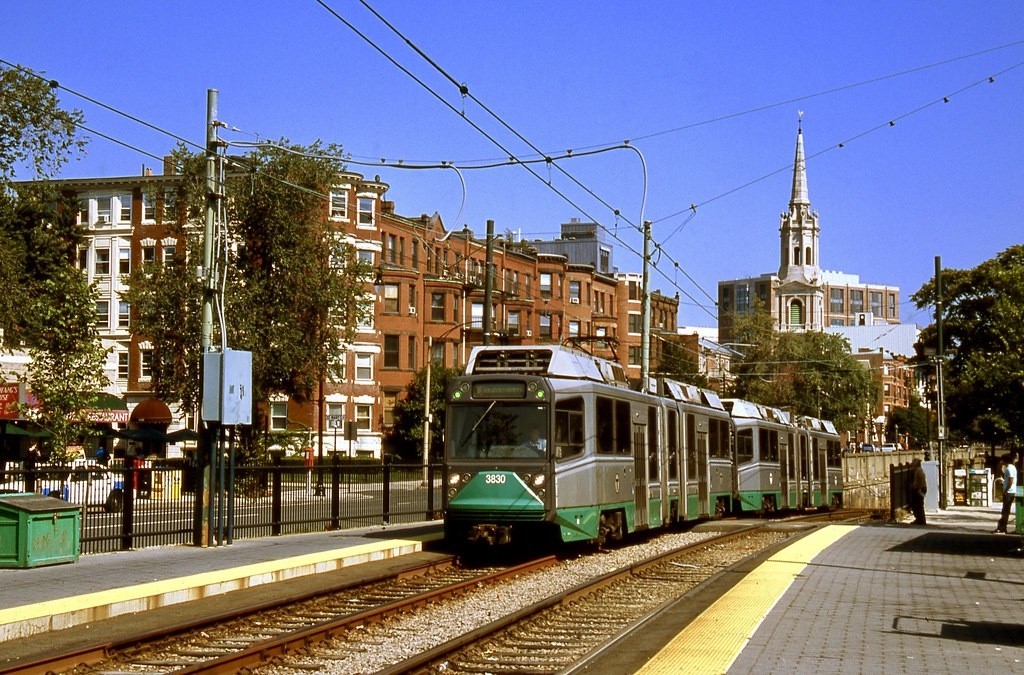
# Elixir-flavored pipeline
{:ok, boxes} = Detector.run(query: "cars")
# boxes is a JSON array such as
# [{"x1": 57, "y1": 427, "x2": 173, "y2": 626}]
[
  {"x1": 0, "y1": 459, "x2": 136, "y2": 514},
  {"x1": 863, "y1": 443, "x2": 873, "y2": 453}
]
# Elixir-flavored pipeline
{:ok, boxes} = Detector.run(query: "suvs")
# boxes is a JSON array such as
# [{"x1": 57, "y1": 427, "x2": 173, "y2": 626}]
[{"x1": 875, "y1": 442, "x2": 903, "y2": 453}]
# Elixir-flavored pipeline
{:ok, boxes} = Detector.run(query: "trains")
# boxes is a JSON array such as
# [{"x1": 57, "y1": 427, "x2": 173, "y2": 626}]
[{"x1": 441, "y1": 345, "x2": 845, "y2": 555}]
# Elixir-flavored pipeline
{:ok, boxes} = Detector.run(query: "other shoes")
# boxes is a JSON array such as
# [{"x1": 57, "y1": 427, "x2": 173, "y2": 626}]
[
  {"x1": 911, "y1": 521, "x2": 925, "y2": 525},
  {"x1": 991, "y1": 528, "x2": 1006, "y2": 534}
]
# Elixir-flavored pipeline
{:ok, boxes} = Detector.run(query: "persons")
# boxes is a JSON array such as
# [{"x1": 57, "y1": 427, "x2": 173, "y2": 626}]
[
  {"x1": 990, "y1": 454, "x2": 1017, "y2": 534},
  {"x1": 911, "y1": 458, "x2": 927, "y2": 524},
  {"x1": 520, "y1": 424, "x2": 546, "y2": 452}
]
